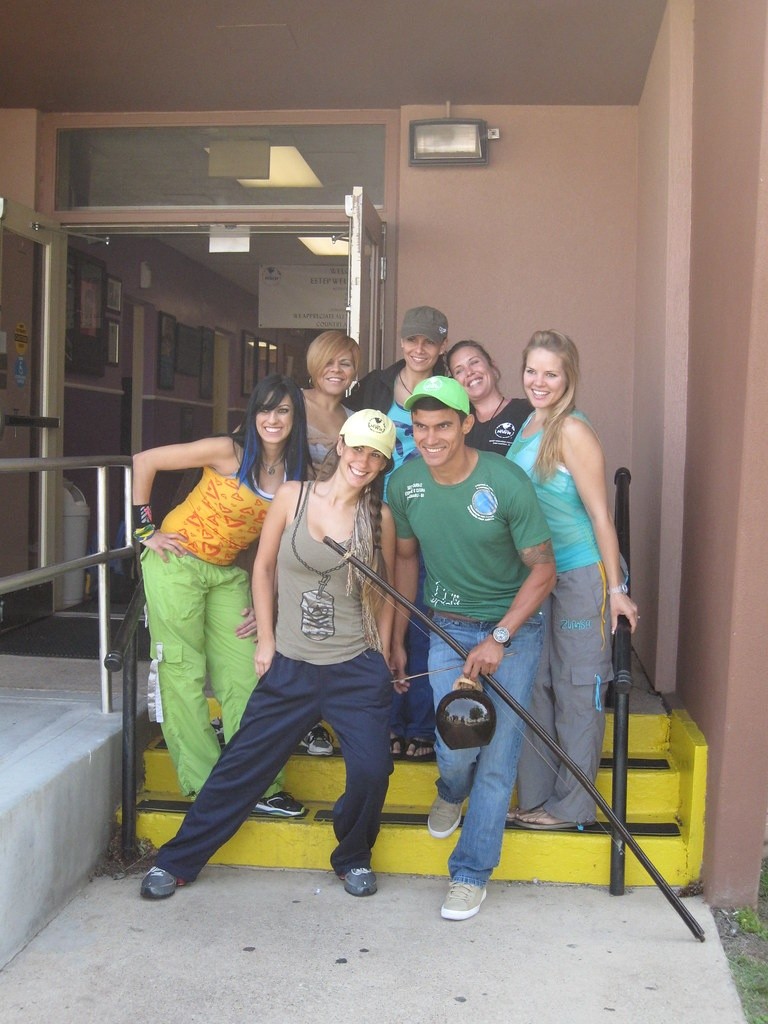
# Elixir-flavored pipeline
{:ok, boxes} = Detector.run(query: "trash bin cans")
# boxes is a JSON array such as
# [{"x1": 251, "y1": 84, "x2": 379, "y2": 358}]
[{"x1": 63, "y1": 478, "x2": 90, "y2": 609}]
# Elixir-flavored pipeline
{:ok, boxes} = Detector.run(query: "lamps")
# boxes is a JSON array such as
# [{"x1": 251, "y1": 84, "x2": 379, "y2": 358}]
[{"x1": 408, "y1": 118, "x2": 489, "y2": 166}]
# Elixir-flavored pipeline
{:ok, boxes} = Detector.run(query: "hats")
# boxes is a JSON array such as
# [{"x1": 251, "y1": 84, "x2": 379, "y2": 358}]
[
  {"x1": 403, "y1": 376, "x2": 470, "y2": 416},
  {"x1": 401, "y1": 305, "x2": 448, "y2": 343},
  {"x1": 339, "y1": 408, "x2": 396, "y2": 460}
]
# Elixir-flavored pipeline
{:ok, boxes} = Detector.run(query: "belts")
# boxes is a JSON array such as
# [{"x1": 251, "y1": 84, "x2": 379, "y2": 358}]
[{"x1": 433, "y1": 610, "x2": 479, "y2": 624}]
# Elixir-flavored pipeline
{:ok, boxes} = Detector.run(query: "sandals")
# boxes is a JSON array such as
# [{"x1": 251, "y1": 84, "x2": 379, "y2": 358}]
[
  {"x1": 513, "y1": 806, "x2": 595, "y2": 829},
  {"x1": 505, "y1": 807, "x2": 520, "y2": 824}
]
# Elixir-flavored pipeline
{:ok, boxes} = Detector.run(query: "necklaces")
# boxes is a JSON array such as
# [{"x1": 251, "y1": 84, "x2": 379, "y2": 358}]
[
  {"x1": 490, "y1": 397, "x2": 504, "y2": 421},
  {"x1": 259, "y1": 460, "x2": 284, "y2": 475},
  {"x1": 399, "y1": 370, "x2": 413, "y2": 396}
]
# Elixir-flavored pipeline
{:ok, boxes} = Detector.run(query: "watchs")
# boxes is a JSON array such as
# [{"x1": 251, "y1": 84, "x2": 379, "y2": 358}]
[
  {"x1": 607, "y1": 583, "x2": 628, "y2": 595},
  {"x1": 490, "y1": 626, "x2": 512, "y2": 648}
]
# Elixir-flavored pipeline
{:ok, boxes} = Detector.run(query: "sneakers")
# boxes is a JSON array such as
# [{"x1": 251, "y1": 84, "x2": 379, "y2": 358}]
[
  {"x1": 441, "y1": 880, "x2": 486, "y2": 920},
  {"x1": 251, "y1": 790, "x2": 305, "y2": 817},
  {"x1": 428, "y1": 795, "x2": 465, "y2": 839},
  {"x1": 300, "y1": 721, "x2": 335, "y2": 756},
  {"x1": 209, "y1": 717, "x2": 226, "y2": 747},
  {"x1": 140, "y1": 865, "x2": 183, "y2": 898},
  {"x1": 338, "y1": 867, "x2": 376, "y2": 896}
]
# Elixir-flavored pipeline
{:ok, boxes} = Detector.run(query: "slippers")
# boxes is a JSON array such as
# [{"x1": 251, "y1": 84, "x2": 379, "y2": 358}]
[
  {"x1": 404, "y1": 736, "x2": 437, "y2": 761},
  {"x1": 388, "y1": 737, "x2": 402, "y2": 760}
]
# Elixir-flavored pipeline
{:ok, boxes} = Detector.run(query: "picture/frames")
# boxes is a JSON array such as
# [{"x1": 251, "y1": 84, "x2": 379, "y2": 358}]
[
  {"x1": 241, "y1": 329, "x2": 278, "y2": 396},
  {"x1": 103, "y1": 273, "x2": 122, "y2": 367},
  {"x1": 158, "y1": 310, "x2": 214, "y2": 400}
]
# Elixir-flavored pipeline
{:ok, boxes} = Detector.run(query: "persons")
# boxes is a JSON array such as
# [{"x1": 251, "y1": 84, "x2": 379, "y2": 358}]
[
  {"x1": 340, "y1": 306, "x2": 446, "y2": 763},
  {"x1": 141, "y1": 409, "x2": 395, "y2": 900},
  {"x1": 390, "y1": 375, "x2": 557, "y2": 920},
  {"x1": 505, "y1": 329, "x2": 638, "y2": 830},
  {"x1": 446, "y1": 340, "x2": 537, "y2": 456},
  {"x1": 210, "y1": 330, "x2": 361, "y2": 755}
]
